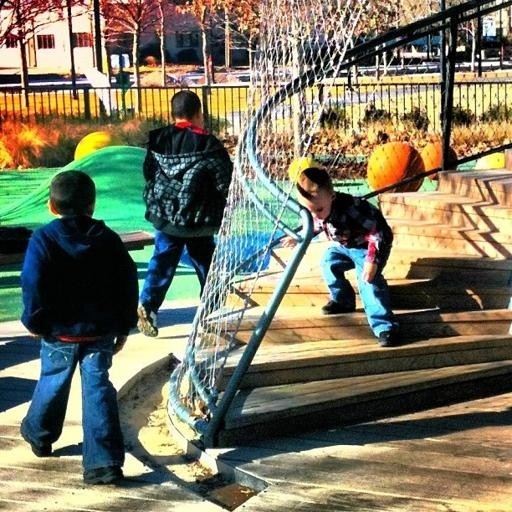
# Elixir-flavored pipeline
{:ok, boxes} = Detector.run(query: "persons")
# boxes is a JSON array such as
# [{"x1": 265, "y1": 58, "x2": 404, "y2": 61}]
[
  {"x1": 17, "y1": 168, "x2": 139, "y2": 487},
  {"x1": 279, "y1": 165, "x2": 403, "y2": 347},
  {"x1": 131, "y1": 89, "x2": 233, "y2": 338}
]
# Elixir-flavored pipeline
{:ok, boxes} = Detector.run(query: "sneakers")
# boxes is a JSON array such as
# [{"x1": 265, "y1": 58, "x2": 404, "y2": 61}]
[
  {"x1": 84, "y1": 465, "x2": 124, "y2": 484},
  {"x1": 20, "y1": 418, "x2": 52, "y2": 456},
  {"x1": 134, "y1": 302, "x2": 159, "y2": 338},
  {"x1": 323, "y1": 301, "x2": 354, "y2": 315},
  {"x1": 381, "y1": 331, "x2": 396, "y2": 348}
]
